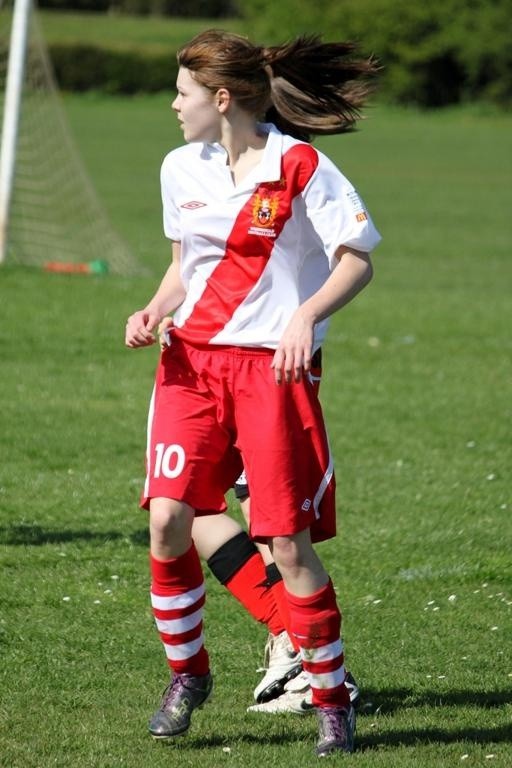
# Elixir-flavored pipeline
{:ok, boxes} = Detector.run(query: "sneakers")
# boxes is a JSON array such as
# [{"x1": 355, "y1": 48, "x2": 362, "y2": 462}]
[
  {"x1": 246, "y1": 631, "x2": 359, "y2": 715},
  {"x1": 149, "y1": 670, "x2": 213, "y2": 740},
  {"x1": 316, "y1": 706, "x2": 356, "y2": 758}
]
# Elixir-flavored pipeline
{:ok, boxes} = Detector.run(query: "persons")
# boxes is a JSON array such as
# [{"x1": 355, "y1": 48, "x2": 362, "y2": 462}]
[
  {"x1": 156, "y1": 100, "x2": 363, "y2": 719},
  {"x1": 122, "y1": 27, "x2": 382, "y2": 758}
]
